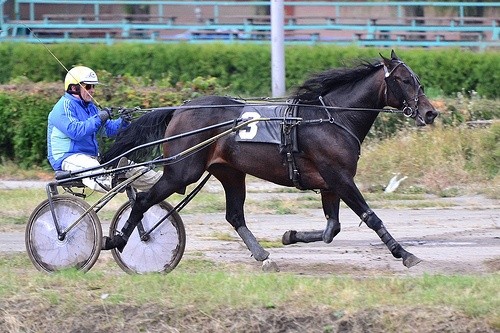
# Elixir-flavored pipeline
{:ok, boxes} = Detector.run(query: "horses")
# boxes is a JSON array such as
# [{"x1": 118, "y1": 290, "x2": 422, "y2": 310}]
[{"x1": 99, "y1": 49, "x2": 437, "y2": 269}]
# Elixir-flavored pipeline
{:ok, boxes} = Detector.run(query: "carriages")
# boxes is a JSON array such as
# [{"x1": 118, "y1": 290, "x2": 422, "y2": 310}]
[{"x1": 24, "y1": 49, "x2": 439, "y2": 277}]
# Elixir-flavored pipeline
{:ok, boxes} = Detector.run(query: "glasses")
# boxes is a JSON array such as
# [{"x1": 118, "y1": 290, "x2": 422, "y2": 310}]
[{"x1": 84, "y1": 84, "x2": 95, "y2": 90}]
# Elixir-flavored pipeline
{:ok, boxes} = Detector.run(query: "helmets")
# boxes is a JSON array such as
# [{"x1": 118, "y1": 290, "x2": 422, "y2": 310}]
[{"x1": 64, "y1": 66, "x2": 98, "y2": 92}]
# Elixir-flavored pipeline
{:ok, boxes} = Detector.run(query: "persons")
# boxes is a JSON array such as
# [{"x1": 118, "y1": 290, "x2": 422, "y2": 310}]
[{"x1": 47, "y1": 65, "x2": 186, "y2": 194}]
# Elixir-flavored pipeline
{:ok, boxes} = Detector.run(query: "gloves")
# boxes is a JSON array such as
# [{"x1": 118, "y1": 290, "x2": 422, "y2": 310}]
[
  {"x1": 118, "y1": 107, "x2": 133, "y2": 121},
  {"x1": 101, "y1": 106, "x2": 114, "y2": 120}
]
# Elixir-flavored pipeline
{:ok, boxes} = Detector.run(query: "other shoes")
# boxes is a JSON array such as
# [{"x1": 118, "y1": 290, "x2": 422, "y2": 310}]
[{"x1": 111, "y1": 157, "x2": 128, "y2": 193}]
